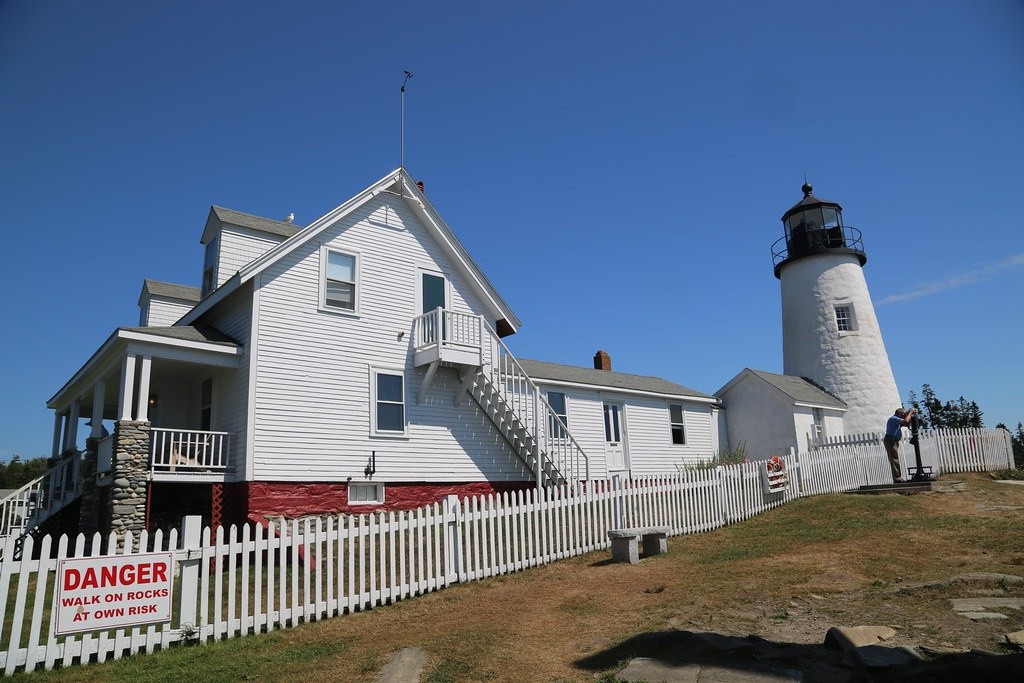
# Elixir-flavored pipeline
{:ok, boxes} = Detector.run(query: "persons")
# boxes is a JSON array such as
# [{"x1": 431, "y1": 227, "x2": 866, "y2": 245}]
[
  {"x1": 85, "y1": 417, "x2": 109, "y2": 438},
  {"x1": 884, "y1": 408, "x2": 918, "y2": 483}
]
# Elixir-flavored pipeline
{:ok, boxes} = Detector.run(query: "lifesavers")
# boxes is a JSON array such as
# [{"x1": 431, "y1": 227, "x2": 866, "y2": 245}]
[{"x1": 769, "y1": 456, "x2": 786, "y2": 487}]
